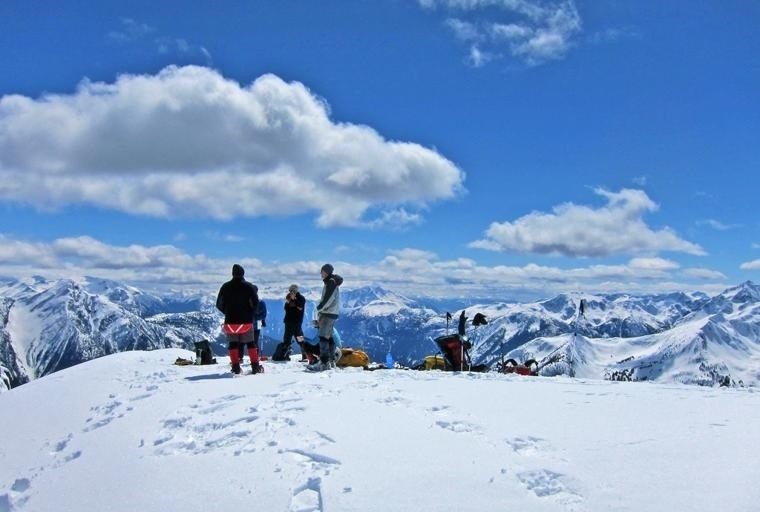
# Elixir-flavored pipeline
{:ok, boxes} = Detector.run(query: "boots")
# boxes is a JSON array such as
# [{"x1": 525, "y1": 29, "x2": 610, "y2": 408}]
[
  {"x1": 231, "y1": 363, "x2": 240, "y2": 373},
  {"x1": 251, "y1": 362, "x2": 263, "y2": 374}
]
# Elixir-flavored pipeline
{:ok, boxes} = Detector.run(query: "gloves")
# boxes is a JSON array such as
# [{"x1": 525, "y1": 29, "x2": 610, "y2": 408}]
[
  {"x1": 472, "y1": 313, "x2": 488, "y2": 326},
  {"x1": 458, "y1": 310, "x2": 467, "y2": 334}
]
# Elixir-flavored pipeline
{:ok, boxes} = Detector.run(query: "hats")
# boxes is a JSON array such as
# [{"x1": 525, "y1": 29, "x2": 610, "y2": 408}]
[
  {"x1": 289, "y1": 284, "x2": 299, "y2": 292},
  {"x1": 232, "y1": 264, "x2": 244, "y2": 275},
  {"x1": 322, "y1": 263, "x2": 334, "y2": 273}
]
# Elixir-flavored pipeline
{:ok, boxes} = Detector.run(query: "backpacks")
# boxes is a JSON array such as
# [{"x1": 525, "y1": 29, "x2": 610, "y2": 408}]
[
  {"x1": 496, "y1": 358, "x2": 538, "y2": 374},
  {"x1": 338, "y1": 348, "x2": 368, "y2": 367},
  {"x1": 272, "y1": 343, "x2": 289, "y2": 361}
]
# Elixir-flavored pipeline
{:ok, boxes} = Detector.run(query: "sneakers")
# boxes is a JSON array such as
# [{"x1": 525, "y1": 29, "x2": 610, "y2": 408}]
[{"x1": 298, "y1": 358, "x2": 336, "y2": 371}]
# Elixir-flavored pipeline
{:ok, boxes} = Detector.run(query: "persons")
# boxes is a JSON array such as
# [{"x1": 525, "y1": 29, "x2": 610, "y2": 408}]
[
  {"x1": 305, "y1": 263, "x2": 344, "y2": 371},
  {"x1": 216, "y1": 263, "x2": 266, "y2": 376},
  {"x1": 238, "y1": 285, "x2": 268, "y2": 363},
  {"x1": 282, "y1": 284, "x2": 309, "y2": 362},
  {"x1": 296, "y1": 310, "x2": 342, "y2": 367}
]
